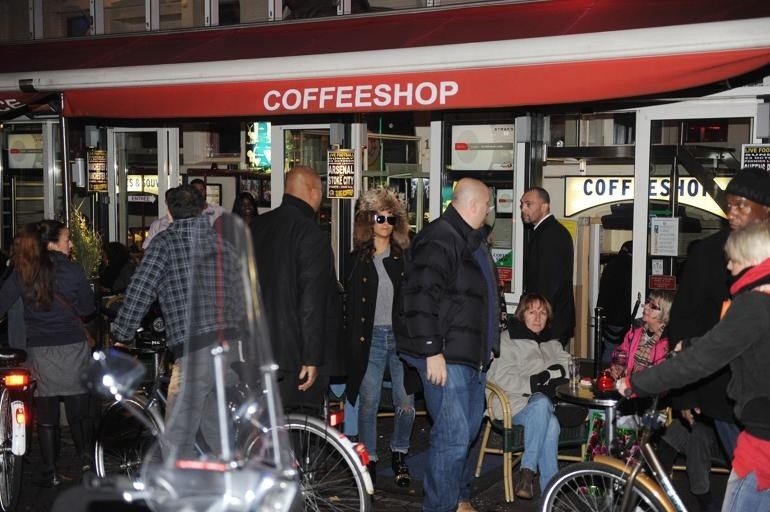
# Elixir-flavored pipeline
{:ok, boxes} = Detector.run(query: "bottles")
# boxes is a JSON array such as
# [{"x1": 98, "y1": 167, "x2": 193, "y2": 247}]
[{"x1": 595, "y1": 370, "x2": 616, "y2": 391}]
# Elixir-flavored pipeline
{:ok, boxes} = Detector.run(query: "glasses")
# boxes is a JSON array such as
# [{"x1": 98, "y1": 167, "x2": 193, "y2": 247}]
[
  {"x1": 646, "y1": 298, "x2": 658, "y2": 310},
  {"x1": 374, "y1": 214, "x2": 397, "y2": 227}
]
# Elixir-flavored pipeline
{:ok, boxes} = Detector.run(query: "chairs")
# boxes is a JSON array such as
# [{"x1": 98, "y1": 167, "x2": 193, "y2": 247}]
[{"x1": 471, "y1": 379, "x2": 590, "y2": 501}]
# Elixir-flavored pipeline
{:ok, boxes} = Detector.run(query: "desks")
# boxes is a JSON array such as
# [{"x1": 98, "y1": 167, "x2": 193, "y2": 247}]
[{"x1": 548, "y1": 378, "x2": 667, "y2": 511}]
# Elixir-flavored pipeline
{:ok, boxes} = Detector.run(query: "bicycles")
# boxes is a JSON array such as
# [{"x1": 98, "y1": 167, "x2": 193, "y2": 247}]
[
  {"x1": 90, "y1": 327, "x2": 375, "y2": 511},
  {"x1": 535, "y1": 350, "x2": 689, "y2": 512},
  {"x1": 0, "y1": 346, "x2": 38, "y2": 511}
]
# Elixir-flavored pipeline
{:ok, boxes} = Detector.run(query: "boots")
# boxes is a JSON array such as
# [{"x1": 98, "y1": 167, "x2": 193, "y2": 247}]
[
  {"x1": 36, "y1": 424, "x2": 73, "y2": 486},
  {"x1": 67, "y1": 416, "x2": 95, "y2": 473}
]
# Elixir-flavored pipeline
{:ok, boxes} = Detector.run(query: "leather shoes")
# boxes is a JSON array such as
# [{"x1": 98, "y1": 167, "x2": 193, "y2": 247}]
[{"x1": 391, "y1": 455, "x2": 411, "y2": 484}]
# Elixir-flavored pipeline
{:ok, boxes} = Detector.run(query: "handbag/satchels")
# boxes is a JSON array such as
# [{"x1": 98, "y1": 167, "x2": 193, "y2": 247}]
[{"x1": 84, "y1": 326, "x2": 95, "y2": 348}]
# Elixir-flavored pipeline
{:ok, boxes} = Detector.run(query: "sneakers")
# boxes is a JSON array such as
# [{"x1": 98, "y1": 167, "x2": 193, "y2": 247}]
[{"x1": 514, "y1": 467, "x2": 535, "y2": 498}]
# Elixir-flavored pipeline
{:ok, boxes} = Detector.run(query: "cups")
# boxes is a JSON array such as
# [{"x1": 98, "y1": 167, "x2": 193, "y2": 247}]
[{"x1": 567, "y1": 356, "x2": 580, "y2": 389}]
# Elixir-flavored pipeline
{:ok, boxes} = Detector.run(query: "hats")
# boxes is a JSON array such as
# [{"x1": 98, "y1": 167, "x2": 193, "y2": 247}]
[{"x1": 724, "y1": 166, "x2": 770, "y2": 207}]
